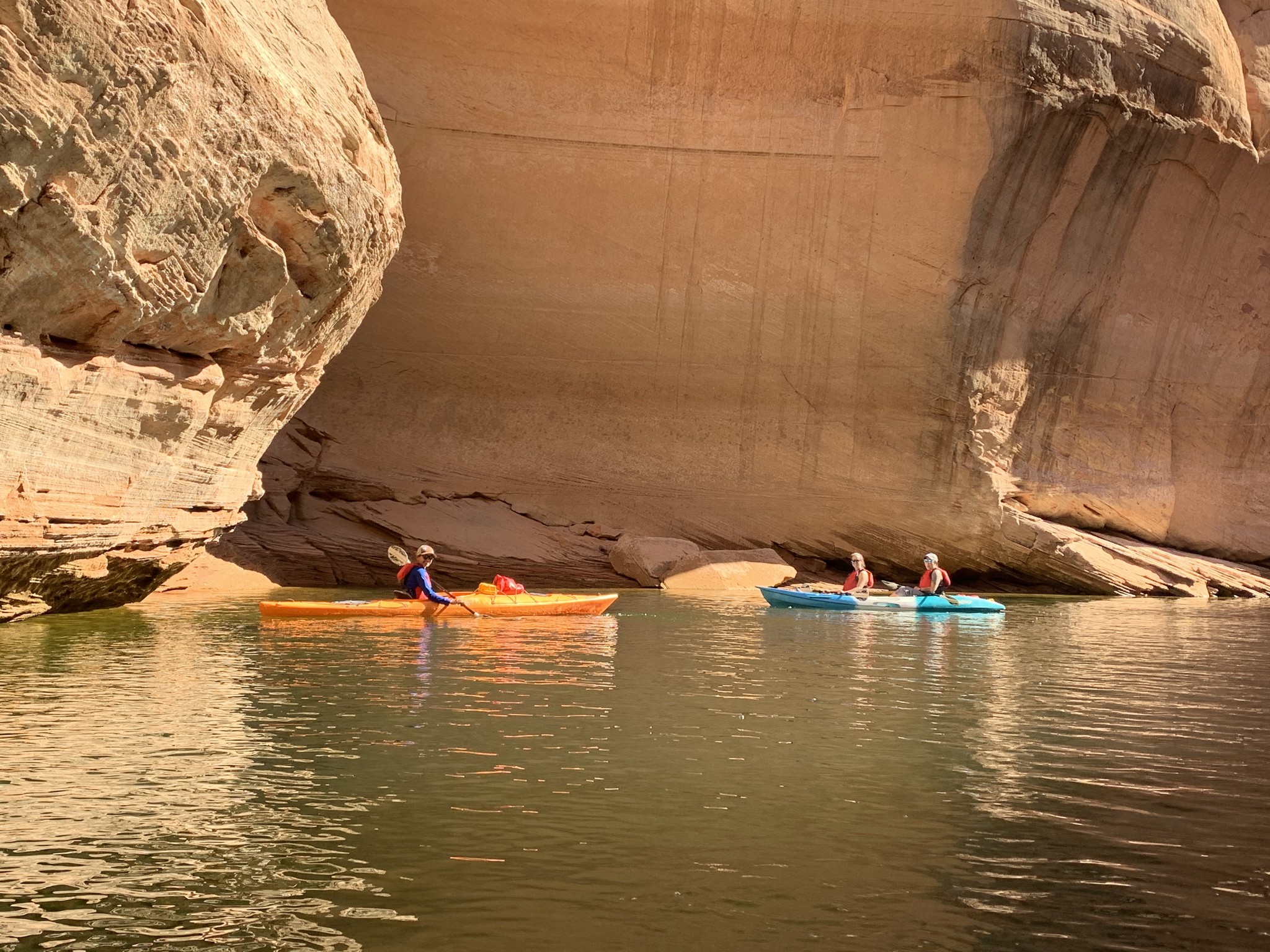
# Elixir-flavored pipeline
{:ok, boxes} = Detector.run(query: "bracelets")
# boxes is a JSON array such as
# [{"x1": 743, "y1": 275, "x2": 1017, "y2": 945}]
[{"x1": 919, "y1": 587, "x2": 921, "y2": 591}]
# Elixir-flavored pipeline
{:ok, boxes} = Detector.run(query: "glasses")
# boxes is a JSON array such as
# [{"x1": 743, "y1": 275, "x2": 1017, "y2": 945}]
[
  {"x1": 422, "y1": 555, "x2": 434, "y2": 560},
  {"x1": 923, "y1": 559, "x2": 934, "y2": 563},
  {"x1": 851, "y1": 559, "x2": 862, "y2": 562}
]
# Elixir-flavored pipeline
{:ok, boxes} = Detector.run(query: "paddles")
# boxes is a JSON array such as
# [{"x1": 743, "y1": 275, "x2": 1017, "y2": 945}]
[
  {"x1": 388, "y1": 543, "x2": 492, "y2": 619},
  {"x1": 811, "y1": 587, "x2": 870, "y2": 602},
  {"x1": 881, "y1": 578, "x2": 960, "y2": 607}
]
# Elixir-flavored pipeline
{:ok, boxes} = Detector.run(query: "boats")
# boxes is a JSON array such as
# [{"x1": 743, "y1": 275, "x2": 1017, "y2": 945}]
[
  {"x1": 754, "y1": 586, "x2": 1006, "y2": 612},
  {"x1": 259, "y1": 583, "x2": 619, "y2": 617}
]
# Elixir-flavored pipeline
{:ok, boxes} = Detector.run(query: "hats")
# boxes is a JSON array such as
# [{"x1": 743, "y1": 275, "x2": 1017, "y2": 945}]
[
  {"x1": 417, "y1": 545, "x2": 437, "y2": 558},
  {"x1": 924, "y1": 553, "x2": 938, "y2": 563}
]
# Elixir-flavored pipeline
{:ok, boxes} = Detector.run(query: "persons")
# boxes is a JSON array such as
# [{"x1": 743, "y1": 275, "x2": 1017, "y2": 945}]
[
  {"x1": 401, "y1": 545, "x2": 465, "y2": 606},
  {"x1": 889, "y1": 553, "x2": 945, "y2": 597},
  {"x1": 796, "y1": 553, "x2": 869, "y2": 601}
]
